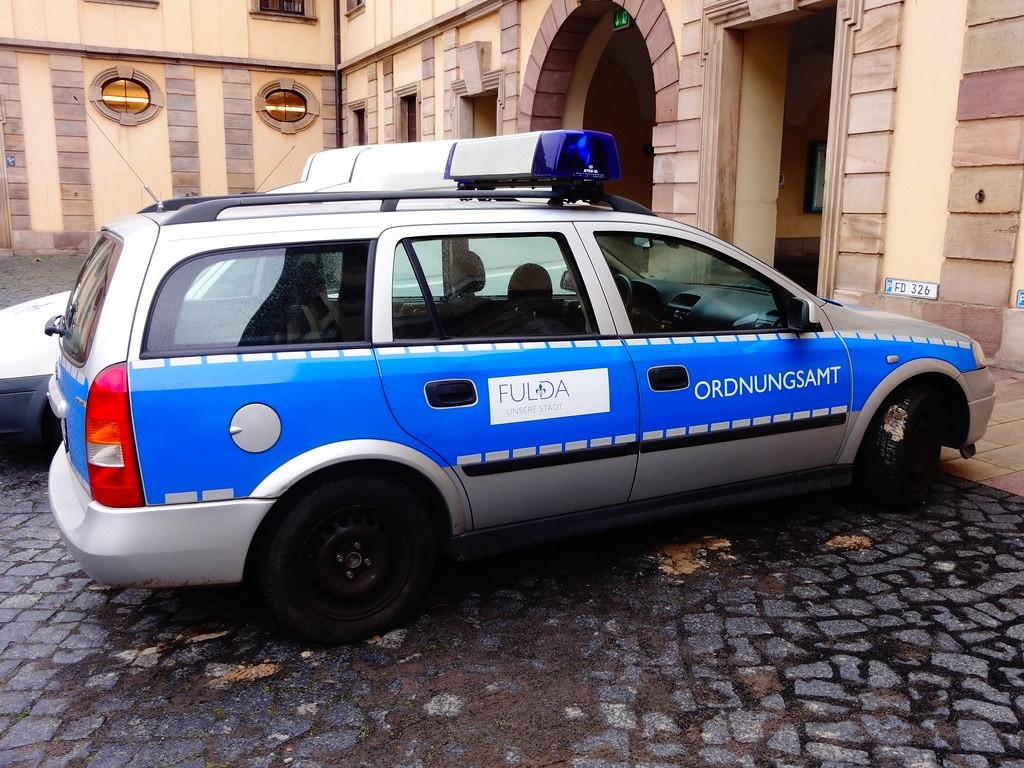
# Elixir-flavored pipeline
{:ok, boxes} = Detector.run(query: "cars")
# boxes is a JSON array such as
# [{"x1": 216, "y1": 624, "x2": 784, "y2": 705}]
[{"x1": 0, "y1": 232, "x2": 577, "y2": 461}]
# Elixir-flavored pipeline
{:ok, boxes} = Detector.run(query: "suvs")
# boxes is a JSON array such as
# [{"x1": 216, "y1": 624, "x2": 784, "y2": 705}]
[{"x1": 43, "y1": 182, "x2": 995, "y2": 645}]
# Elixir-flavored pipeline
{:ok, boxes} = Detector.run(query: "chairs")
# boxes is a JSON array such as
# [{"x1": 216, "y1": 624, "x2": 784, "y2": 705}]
[
  {"x1": 438, "y1": 251, "x2": 491, "y2": 325},
  {"x1": 495, "y1": 264, "x2": 568, "y2": 330}
]
